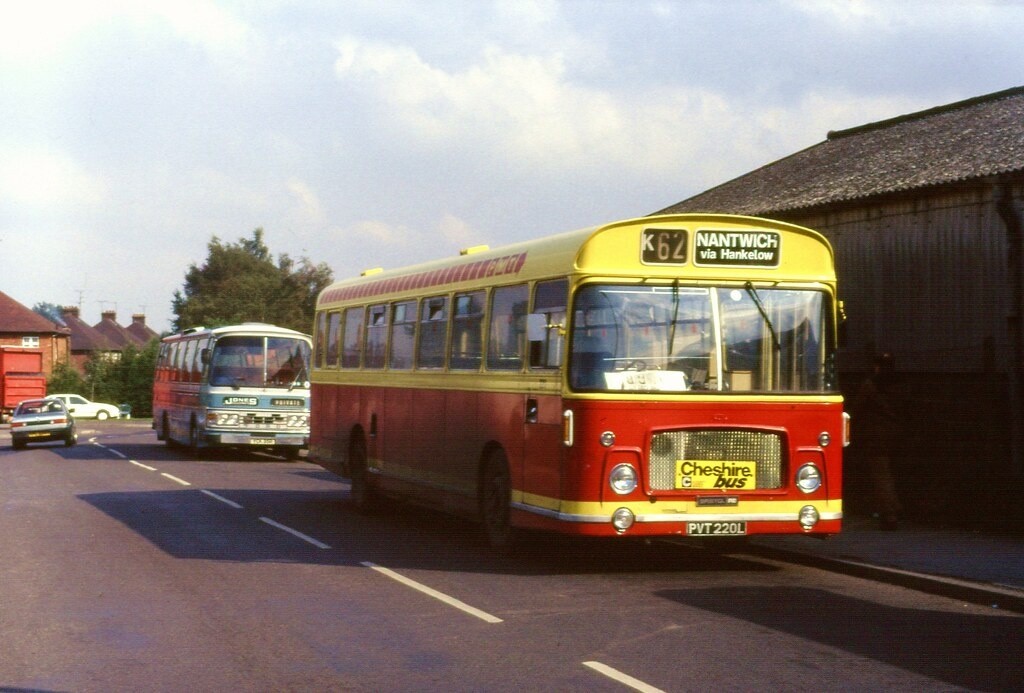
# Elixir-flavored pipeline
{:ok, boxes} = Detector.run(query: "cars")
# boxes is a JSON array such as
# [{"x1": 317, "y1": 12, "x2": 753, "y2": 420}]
[
  {"x1": 28, "y1": 394, "x2": 121, "y2": 421},
  {"x1": 9, "y1": 398, "x2": 77, "y2": 448}
]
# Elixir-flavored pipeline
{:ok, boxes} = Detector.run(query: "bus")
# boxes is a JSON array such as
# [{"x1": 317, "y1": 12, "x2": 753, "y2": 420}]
[
  {"x1": 306, "y1": 216, "x2": 852, "y2": 549},
  {"x1": 153, "y1": 322, "x2": 312, "y2": 454}
]
